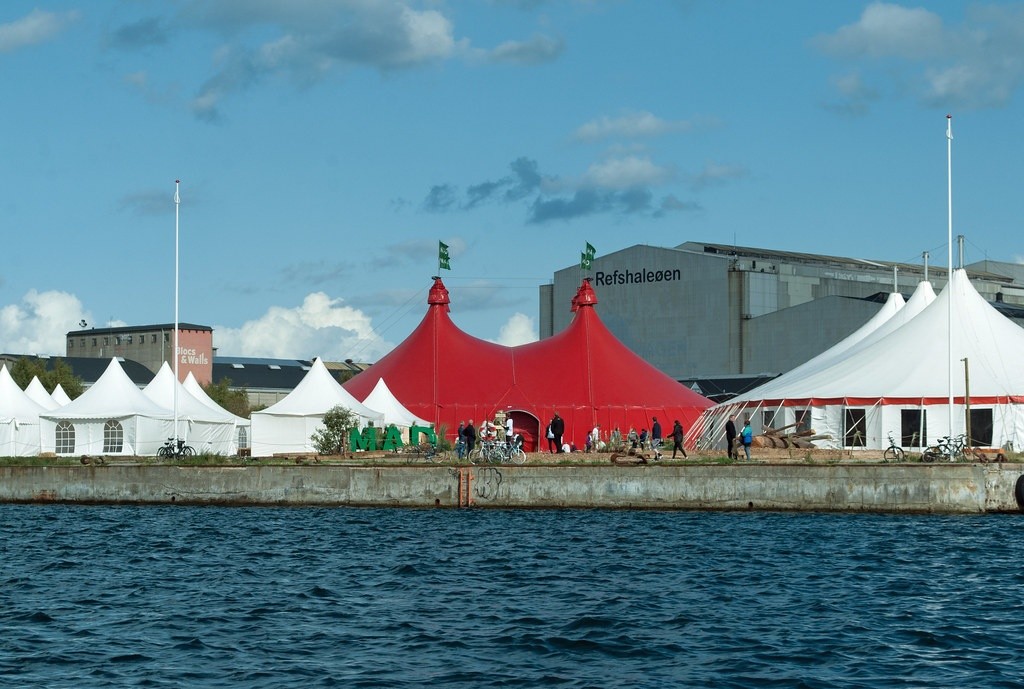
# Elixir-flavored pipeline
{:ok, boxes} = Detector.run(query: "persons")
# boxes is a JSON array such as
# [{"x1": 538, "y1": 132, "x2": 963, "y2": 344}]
[
  {"x1": 667, "y1": 420, "x2": 688, "y2": 460},
  {"x1": 651, "y1": 416, "x2": 663, "y2": 461},
  {"x1": 587, "y1": 424, "x2": 650, "y2": 453},
  {"x1": 741, "y1": 419, "x2": 753, "y2": 461},
  {"x1": 545, "y1": 412, "x2": 565, "y2": 454},
  {"x1": 561, "y1": 435, "x2": 578, "y2": 454},
  {"x1": 726, "y1": 415, "x2": 739, "y2": 460},
  {"x1": 513, "y1": 431, "x2": 526, "y2": 454},
  {"x1": 458, "y1": 419, "x2": 476, "y2": 460},
  {"x1": 502, "y1": 413, "x2": 514, "y2": 461}
]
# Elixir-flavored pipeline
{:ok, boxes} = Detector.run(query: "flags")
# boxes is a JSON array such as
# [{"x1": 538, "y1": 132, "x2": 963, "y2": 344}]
[
  {"x1": 581, "y1": 252, "x2": 592, "y2": 271},
  {"x1": 586, "y1": 242, "x2": 596, "y2": 261},
  {"x1": 439, "y1": 241, "x2": 450, "y2": 270}
]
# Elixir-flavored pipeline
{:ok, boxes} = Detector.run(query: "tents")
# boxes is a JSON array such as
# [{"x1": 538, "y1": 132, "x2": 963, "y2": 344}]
[
  {"x1": 702, "y1": 270, "x2": 1024, "y2": 457},
  {"x1": 251, "y1": 357, "x2": 434, "y2": 457},
  {"x1": 0, "y1": 357, "x2": 251, "y2": 456}
]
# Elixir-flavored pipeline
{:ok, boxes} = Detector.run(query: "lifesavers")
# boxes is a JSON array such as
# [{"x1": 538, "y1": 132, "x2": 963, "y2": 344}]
[
  {"x1": 1015, "y1": 474, "x2": 1024, "y2": 506},
  {"x1": 479, "y1": 422, "x2": 497, "y2": 442}
]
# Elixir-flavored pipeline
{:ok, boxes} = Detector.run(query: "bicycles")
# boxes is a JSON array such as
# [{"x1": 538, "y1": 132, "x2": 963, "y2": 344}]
[
  {"x1": 156, "y1": 437, "x2": 197, "y2": 465},
  {"x1": 883, "y1": 431, "x2": 905, "y2": 463},
  {"x1": 921, "y1": 433, "x2": 975, "y2": 463},
  {"x1": 449, "y1": 432, "x2": 527, "y2": 466},
  {"x1": 406, "y1": 442, "x2": 447, "y2": 465}
]
